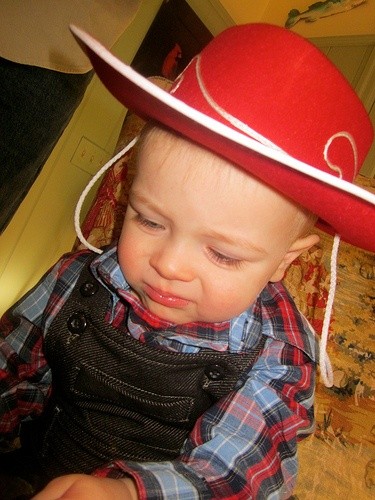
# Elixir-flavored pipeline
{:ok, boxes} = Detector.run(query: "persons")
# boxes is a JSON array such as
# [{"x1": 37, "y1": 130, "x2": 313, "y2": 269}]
[{"x1": 0, "y1": 22, "x2": 375, "y2": 500}]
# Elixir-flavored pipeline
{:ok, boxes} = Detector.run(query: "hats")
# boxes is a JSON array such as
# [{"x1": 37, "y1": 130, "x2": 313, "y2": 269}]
[{"x1": 62, "y1": 21, "x2": 375, "y2": 252}]
